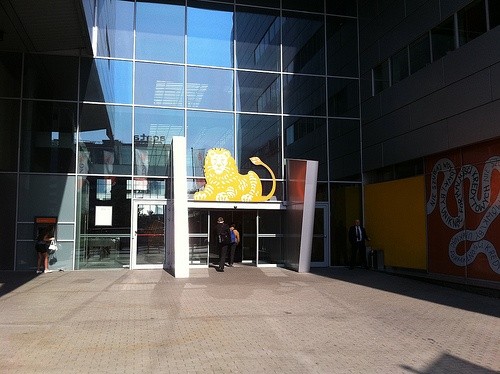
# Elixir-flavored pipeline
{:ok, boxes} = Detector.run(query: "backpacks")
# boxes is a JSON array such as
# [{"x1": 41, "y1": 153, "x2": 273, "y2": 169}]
[{"x1": 229, "y1": 229, "x2": 235, "y2": 243}]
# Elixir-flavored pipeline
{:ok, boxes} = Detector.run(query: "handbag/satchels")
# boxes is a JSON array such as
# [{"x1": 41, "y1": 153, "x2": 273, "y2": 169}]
[
  {"x1": 218, "y1": 234, "x2": 229, "y2": 243},
  {"x1": 49, "y1": 241, "x2": 58, "y2": 250}
]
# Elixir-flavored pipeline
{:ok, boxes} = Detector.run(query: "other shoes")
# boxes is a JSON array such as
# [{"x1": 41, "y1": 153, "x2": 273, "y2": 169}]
[
  {"x1": 44, "y1": 270, "x2": 53, "y2": 273},
  {"x1": 219, "y1": 267, "x2": 224, "y2": 271},
  {"x1": 36, "y1": 271, "x2": 42, "y2": 273},
  {"x1": 229, "y1": 264, "x2": 232, "y2": 267}
]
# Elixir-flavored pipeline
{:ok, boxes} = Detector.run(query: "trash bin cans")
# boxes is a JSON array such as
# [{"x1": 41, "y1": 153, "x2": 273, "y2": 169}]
[
  {"x1": 376, "y1": 249, "x2": 384, "y2": 270},
  {"x1": 368, "y1": 249, "x2": 378, "y2": 271}
]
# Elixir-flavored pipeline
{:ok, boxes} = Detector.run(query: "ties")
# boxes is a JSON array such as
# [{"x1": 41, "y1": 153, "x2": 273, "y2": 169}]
[{"x1": 357, "y1": 226, "x2": 361, "y2": 240}]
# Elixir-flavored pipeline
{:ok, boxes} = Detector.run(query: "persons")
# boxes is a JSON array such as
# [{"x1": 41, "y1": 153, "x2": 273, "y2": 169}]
[
  {"x1": 212, "y1": 217, "x2": 240, "y2": 271},
  {"x1": 36, "y1": 226, "x2": 54, "y2": 273},
  {"x1": 348, "y1": 220, "x2": 371, "y2": 268}
]
